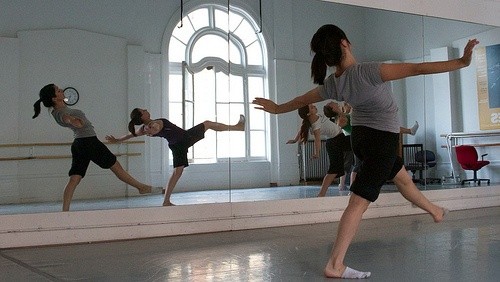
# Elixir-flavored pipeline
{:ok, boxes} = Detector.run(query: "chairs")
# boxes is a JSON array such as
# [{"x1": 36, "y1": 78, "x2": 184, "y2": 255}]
[
  {"x1": 403, "y1": 144, "x2": 428, "y2": 186},
  {"x1": 455, "y1": 145, "x2": 491, "y2": 187},
  {"x1": 415, "y1": 150, "x2": 441, "y2": 185}
]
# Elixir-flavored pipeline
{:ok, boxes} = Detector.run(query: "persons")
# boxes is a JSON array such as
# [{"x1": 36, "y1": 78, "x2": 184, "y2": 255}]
[
  {"x1": 338, "y1": 152, "x2": 351, "y2": 191},
  {"x1": 106, "y1": 108, "x2": 245, "y2": 206},
  {"x1": 322, "y1": 101, "x2": 419, "y2": 186},
  {"x1": 252, "y1": 23, "x2": 479, "y2": 279},
  {"x1": 286, "y1": 104, "x2": 351, "y2": 197},
  {"x1": 32, "y1": 83, "x2": 163, "y2": 211}
]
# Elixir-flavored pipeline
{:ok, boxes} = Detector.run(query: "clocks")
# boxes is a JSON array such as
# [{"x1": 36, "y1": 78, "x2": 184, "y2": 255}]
[{"x1": 62, "y1": 87, "x2": 79, "y2": 106}]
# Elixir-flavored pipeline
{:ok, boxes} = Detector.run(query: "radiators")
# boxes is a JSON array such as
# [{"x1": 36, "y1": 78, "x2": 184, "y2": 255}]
[{"x1": 299, "y1": 139, "x2": 329, "y2": 186}]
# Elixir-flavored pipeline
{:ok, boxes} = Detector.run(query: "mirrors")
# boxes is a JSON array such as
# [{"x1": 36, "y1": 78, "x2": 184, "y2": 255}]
[{"x1": 0, "y1": 0, "x2": 500, "y2": 215}]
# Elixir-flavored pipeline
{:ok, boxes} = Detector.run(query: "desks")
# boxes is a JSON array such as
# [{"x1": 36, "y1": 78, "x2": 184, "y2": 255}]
[{"x1": 440, "y1": 130, "x2": 500, "y2": 186}]
